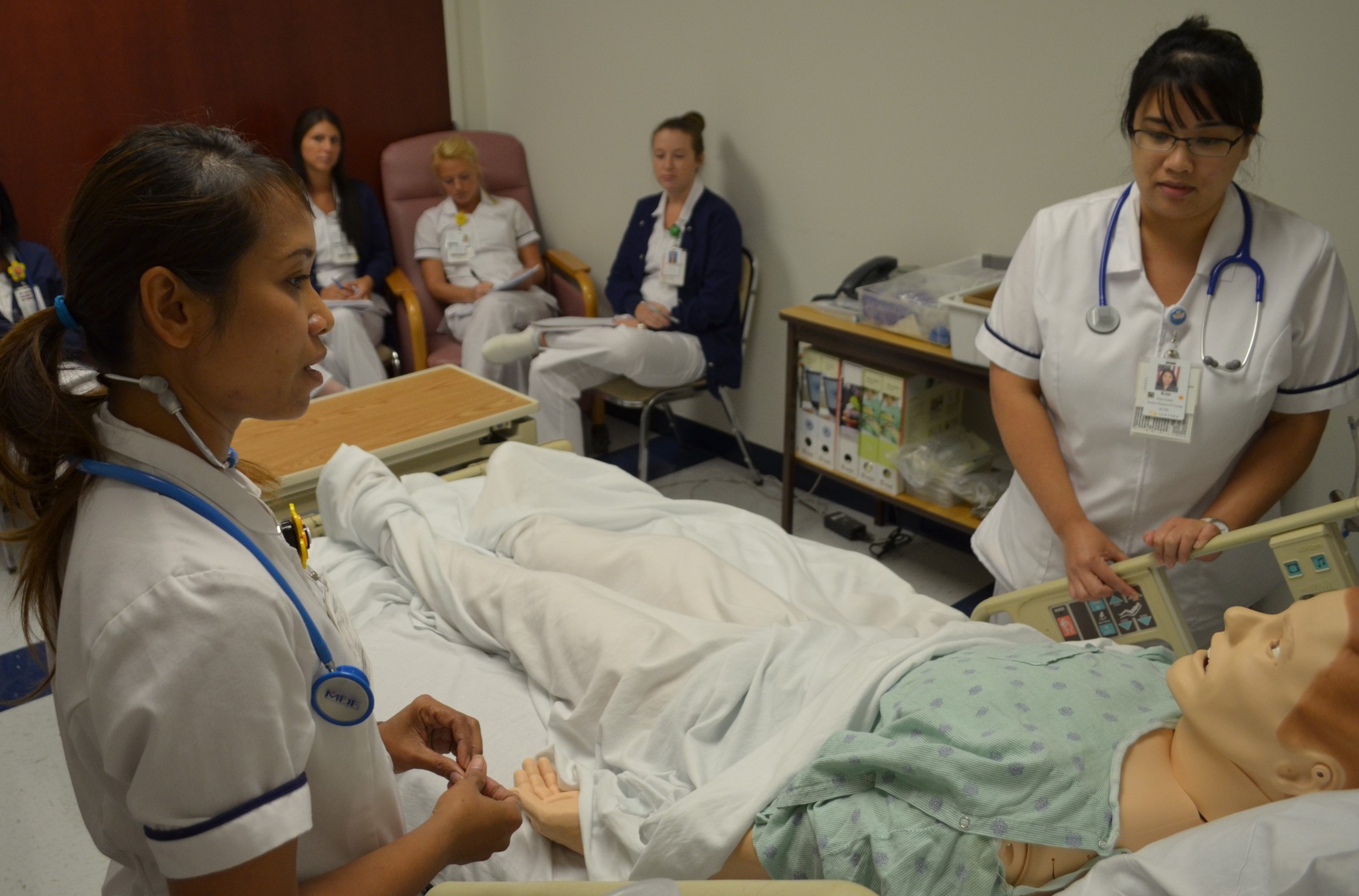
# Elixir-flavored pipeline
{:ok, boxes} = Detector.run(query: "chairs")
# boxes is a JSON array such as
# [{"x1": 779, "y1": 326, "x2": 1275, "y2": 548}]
[
  {"x1": 601, "y1": 247, "x2": 766, "y2": 493},
  {"x1": 379, "y1": 128, "x2": 597, "y2": 381}
]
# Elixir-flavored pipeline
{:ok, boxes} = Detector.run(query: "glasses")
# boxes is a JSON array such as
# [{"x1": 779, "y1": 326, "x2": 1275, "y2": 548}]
[{"x1": 1127, "y1": 119, "x2": 1251, "y2": 156}]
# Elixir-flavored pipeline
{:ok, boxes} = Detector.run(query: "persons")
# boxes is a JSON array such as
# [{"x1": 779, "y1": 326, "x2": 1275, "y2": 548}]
[
  {"x1": 0, "y1": 122, "x2": 524, "y2": 896},
  {"x1": 281, "y1": 106, "x2": 398, "y2": 396},
  {"x1": 480, "y1": 108, "x2": 747, "y2": 461},
  {"x1": 411, "y1": 131, "x2": 561, "y2": 395},
  {"x1": 1153, "y1": 370, "x2": 1179, "y2": 393},
  {"x1": 963, "y1": 7, "x2": 1358, "y2": 635},
  {"x1": 323, "y1": 431, "x2": 1359, "y2": 894},
  {"x1": 0, "y1": 191, "x2": 62, "y2": 332}
]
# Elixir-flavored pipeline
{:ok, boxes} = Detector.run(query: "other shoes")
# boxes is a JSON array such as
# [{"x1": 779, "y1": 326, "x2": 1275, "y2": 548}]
[{"x1": 482, "y1": 324, "x2": 544, "y2": 365}]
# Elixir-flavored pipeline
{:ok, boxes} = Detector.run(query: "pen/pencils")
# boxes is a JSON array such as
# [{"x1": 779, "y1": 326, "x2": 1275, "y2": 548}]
[
  {"x1": 469, "y1": 269, "x2": 484, "y2": 284},
  {"x1": 331, "y1": 278, "x2": 344, "y2": 289},
  {"x1": 640, "y1": 301, "x2": 679, "y2": 324}
]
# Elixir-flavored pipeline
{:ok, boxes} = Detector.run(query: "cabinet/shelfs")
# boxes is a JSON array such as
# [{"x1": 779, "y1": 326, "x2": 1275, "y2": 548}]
[{"x1": 781, "y1": 306, "x2": 1016, "y2": 538}]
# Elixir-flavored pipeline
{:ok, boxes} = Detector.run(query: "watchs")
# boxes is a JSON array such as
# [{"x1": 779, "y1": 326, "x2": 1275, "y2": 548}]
[{"x1": 1201, "y1": 518, "x2": 1231, "y2": 535}]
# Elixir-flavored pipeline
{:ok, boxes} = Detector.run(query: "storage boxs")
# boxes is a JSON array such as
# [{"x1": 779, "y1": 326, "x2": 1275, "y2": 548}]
[
  {"x1": 940, "y1": 280, "x2": 988, "y2": 370},
  {"x1": 858, "y1": 250, "x2": 1012, "y2": 349}
]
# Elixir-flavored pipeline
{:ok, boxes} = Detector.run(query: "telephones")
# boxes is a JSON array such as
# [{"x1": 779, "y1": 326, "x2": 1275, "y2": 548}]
[{"x1": 811, "y1": 255, "x2": 924, "y2": 301}]
